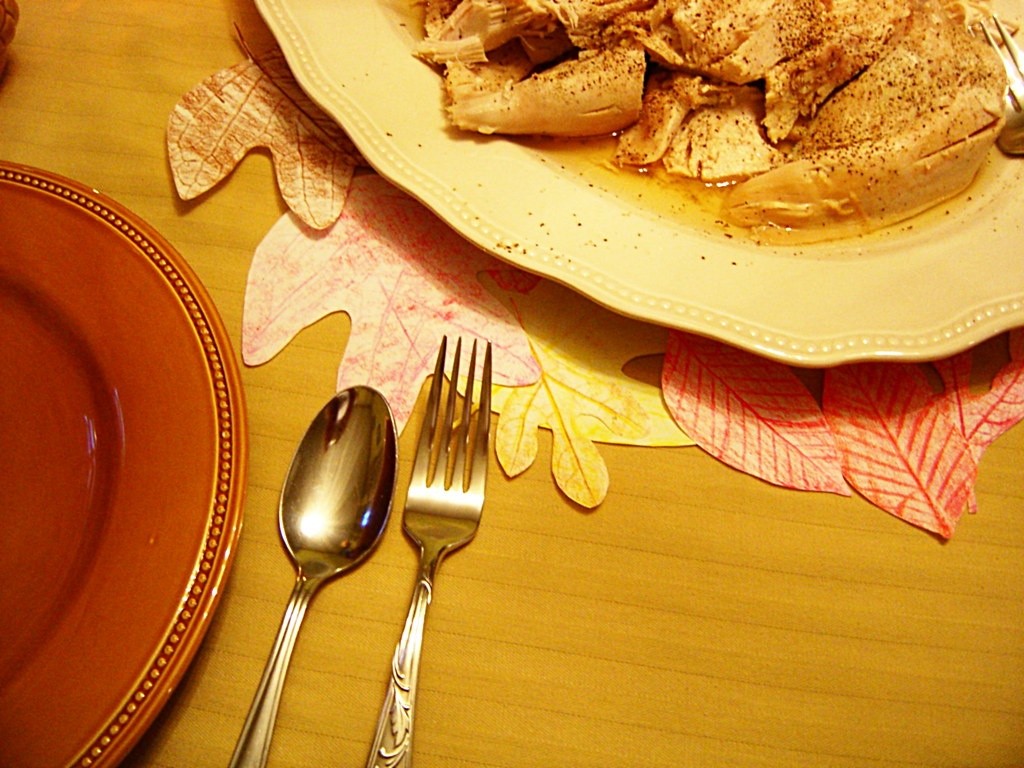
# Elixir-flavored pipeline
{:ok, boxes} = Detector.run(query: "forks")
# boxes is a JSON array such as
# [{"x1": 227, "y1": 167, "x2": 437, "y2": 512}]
[
  {"x1": 367, "y1": 335, "x2": 492, "y2": 768},
  {"x1": 968, "y1": 16, "x2": 1024, "y2": 158}
]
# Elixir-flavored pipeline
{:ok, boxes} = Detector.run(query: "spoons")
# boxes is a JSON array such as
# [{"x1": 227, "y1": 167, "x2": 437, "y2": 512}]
[{"x1": 228, "y1": 385, "x2": 398, "y2": 768}]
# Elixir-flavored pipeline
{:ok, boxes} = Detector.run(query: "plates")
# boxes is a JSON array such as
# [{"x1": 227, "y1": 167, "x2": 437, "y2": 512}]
[
  {"x1": 0, "y1": 161, "x2": 247, "y2": 768},
  {"x1": 254, "y1": 1, "x2": 1024, "y2": 368}
]
